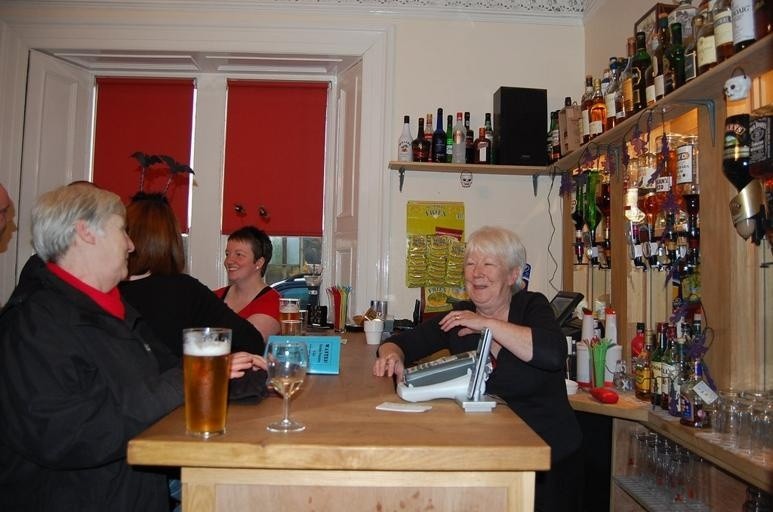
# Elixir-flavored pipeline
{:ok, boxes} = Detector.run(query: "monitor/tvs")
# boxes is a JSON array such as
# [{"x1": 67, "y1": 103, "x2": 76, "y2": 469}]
[{"x1": 549, "y1": 290, "x2": 584, "y2": 325}]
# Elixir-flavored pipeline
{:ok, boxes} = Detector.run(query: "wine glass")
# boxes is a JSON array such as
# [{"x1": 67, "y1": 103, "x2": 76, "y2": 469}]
[{"x1": 264, "y1": 341, "x2": 309, "y2": 433}]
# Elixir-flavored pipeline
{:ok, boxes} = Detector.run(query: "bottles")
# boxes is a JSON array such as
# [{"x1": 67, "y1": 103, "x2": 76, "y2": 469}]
[
  {"x1": 576, "y1": 268, "x2": 709, "y2": 431},
  {"x1": 369, "y1": 300, "x2": 386, "y2": 319},
  {"x1": 557, "y1": 132, "x2": 698, "y2": 266},
  {"x1": 392, "y1": 108, "x2": 496, "y2": 164},
  {"x1": 546, "y1": 0, "x2": 773, "y2": 164},
  {"x1": 721, "y1": 112, "x2": 772, "y2": 245}
]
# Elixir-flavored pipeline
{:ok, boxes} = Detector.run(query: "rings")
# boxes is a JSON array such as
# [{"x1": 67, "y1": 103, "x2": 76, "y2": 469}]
[{"x1": 453, "y1": 315, "x2": 460, "y2": 320}]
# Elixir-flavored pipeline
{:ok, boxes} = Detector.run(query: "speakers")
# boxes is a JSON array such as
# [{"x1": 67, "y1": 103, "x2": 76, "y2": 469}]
[{"x1": 493, "y1": 86, "x2": 548, "y2": 165}]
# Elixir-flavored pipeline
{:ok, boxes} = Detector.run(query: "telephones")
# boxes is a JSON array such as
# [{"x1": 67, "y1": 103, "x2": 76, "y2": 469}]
[{"x1": 456, "y1": 327, "x2": 497, "y2": 412}]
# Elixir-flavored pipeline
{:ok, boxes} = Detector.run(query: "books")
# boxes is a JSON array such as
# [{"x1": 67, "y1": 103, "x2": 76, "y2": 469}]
[{"x1": 263, "y1": 335, "x2": 341, "y2": 375}]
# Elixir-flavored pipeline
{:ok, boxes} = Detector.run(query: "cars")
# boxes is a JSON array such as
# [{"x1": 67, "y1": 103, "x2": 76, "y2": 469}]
[{"x1": 270, "y1": 271, "x2": 321, "y2": 310}]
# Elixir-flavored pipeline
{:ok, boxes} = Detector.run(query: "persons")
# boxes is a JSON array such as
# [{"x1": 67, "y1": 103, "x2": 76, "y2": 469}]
[
  {"x1": 211, "y1": 225, "x2": 284, "y2": 345},
  {"x1": 373, "y1": 226, "x2": 585, "y2": 512},
  {"x1": 0, "y1": 181, "x2": 101, "y2": 286},
  {"x1": 0, "y1": 185, "x2": 269, "y2": 512},
  {"x1": 118, "y1": 191, "x2": 265, "y2": 362}
]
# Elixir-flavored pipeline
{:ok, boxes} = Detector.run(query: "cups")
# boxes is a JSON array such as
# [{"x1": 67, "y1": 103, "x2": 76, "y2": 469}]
[
  {"x1": 278, "y1": 297, "x2": 308, "y2": 335},
  {"x1": 310, "y1": 305, "x2": 326, "y2": 328},
  {"x1": 181, "y1": 325, "x2": 232, "y2": 436},
  {"x1": 363, "y1": 320, "x2": 382, "y2": 345},
  {"x1": 742, "y1": 487, "x2": 772, "y2": 511},
  {"x1": 620, "y1": 433, "x2": 707, "y2": 512},
  {"x1": 710, "y1": 385, "x2": 773, "y2": 461},
  {"x1": 326, "y1": 286, "x2": 350, "y2": 334}
]
